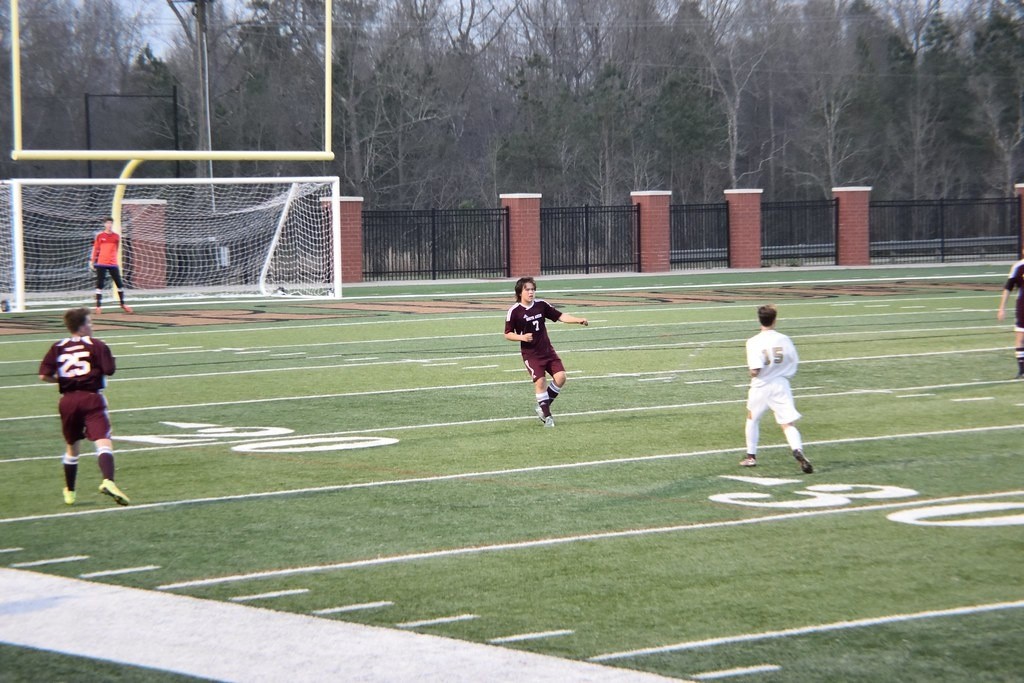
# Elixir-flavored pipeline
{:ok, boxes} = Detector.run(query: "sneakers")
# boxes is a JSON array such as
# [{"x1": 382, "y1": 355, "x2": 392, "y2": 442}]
[
  {"x1": 793, "y1": 449, "x2": 812, "y2": 473},
  {"x1": 545, "y1": 415, "x2": 555, "y2": 428},
  {"x1": 98, "y1": 478, "x2": 130, "y2": 506},
  {"x1": 739, "y1": 457, "x2": 756, "y2": 466},
  {"x1": 536, "y1": 406, "x2": 545, "y2": 422},
  {"x1": 63, "y1": 485, "x2": 76, "y2": 505}
]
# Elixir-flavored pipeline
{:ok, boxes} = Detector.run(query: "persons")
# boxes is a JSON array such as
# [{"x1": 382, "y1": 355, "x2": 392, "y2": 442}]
[
  {"x1": 996, "y1": 257, "x2": 1024, "y2": 380},
  {"x1": 739, "y1": 304, "x2": 813, "y2": 474},
  {"x1": 88, "y1": 217, "x2": 132, "y2": 315},
  {"x1": 503, "y1": 276, "x2": 588, "y2": 428},
  {"x1": 39, "y1": 307, "x2": 131, "y2": 506}
]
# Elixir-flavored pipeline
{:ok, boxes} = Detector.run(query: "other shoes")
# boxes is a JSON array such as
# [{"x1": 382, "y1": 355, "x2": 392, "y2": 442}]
[
  {"x1": 1013, "y1": 372, "x2": 1024, "y2": 378},
  {"x1": 121, "y1": 305, "x2": 132, "y2": 314},
  {"x1": 96, "y1": 307, "x2": 101, "y2": 315}
]
